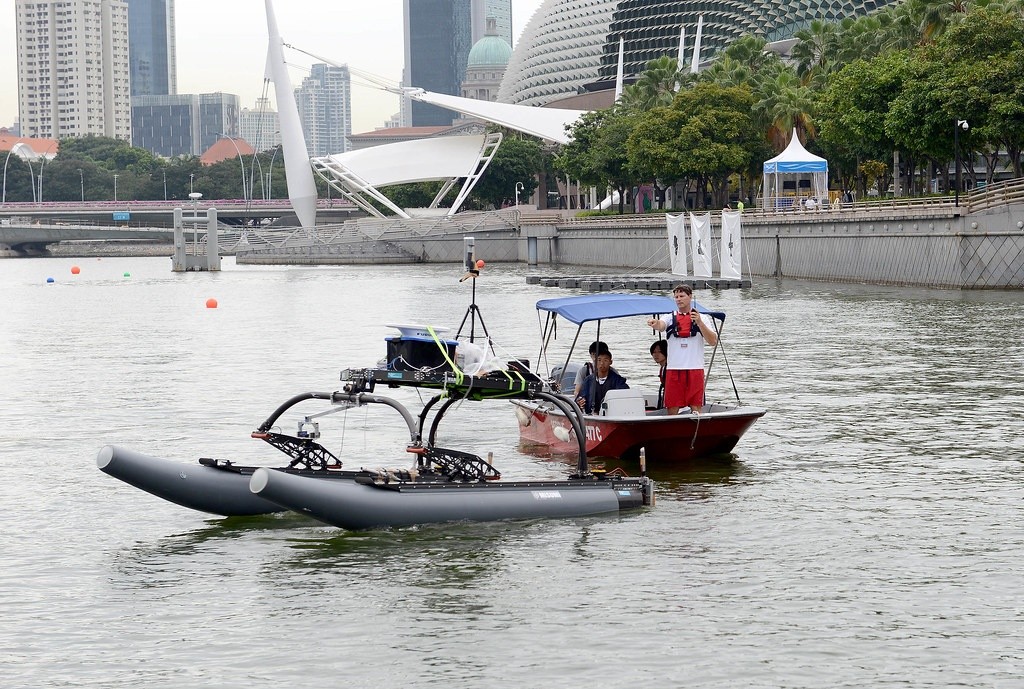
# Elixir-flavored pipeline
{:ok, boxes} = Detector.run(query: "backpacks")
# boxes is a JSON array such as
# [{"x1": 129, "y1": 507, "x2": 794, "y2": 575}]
[{"x1": 847, "y1": 193, "x2": 852, "y2": 202}]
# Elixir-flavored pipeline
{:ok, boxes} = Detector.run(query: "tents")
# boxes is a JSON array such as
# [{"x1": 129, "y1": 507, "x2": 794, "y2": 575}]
[{"x1": 763, "y1": 126, "x2": 828, "y2": 207}]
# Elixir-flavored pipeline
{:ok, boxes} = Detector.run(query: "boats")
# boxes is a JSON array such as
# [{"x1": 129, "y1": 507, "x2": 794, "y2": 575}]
[
  {"x1": 508, "y1": 294, "x2": 767, "y2": 467},
  {"x1": 94, "y1": 236, "x2": 654, "y2": 532}
]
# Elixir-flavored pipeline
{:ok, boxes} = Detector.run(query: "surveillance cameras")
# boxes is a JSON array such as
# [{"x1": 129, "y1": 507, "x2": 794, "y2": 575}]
[{"x1": 962, "y1": 123, "x2": 969, "y2": 130}]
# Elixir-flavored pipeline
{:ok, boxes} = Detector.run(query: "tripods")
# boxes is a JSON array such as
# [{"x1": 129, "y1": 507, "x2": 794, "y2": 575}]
[{"x1": 455, "y1": 278, "x2": 496, "y2": 357}]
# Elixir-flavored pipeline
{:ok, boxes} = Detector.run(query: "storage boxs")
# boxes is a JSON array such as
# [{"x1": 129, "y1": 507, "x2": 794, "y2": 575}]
[{"x1": 385, "y1": 336, "x2": 459, "y2": 369}]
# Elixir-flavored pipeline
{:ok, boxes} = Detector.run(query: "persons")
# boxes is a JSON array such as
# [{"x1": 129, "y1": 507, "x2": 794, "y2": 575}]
[
  {"x1": 578, "y1": 204, "x2": 581, "y2": 209},
  {"x1": 574, "y1": 341, "x2": 619, "y2": 396},
  {"x1": 737, "y1": 199, "x2": 744, "y2": 213},
  {"x1": 834, "y1": 196, "x2": 841, "y2": 209},
  {"x1": 586, "y1": 203, "x2": 591, "y2": 209},
  {"x1": 842, "y1": 192, "x2": 853, "y2": 208},
  {"x1": 647, "y1": 285, "x2": 718, "y2": 415},
  {"x1": 805, "y1": 195, "x2": 815, "y2": 215},
  {"x1": 649, "y1": 339, "x2": 668, "y2": 387},
  {"x1": 574, "y1": 350, "x2": 630, "y2": 415}
]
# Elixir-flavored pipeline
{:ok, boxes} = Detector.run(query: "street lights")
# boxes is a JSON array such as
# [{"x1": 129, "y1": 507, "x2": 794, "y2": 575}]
[
  {"x1": 189, "y1": 174, "x2": 194, "y2": 201},
  {"x1": 516, "y1": 182, "x2": 525, "y2": 209},
  {"x1": 76, "y1": 168, "x2": 84, "y2": 202},
  {"x1": 2, "y1": 139, "x2": 61, "y2": 205},
  {"x1": 214, "y1": 131, "x2": 282, "y2": 204},
  {"x1": 956, "y1": 118, "x2": 969, "y2": 208},
  {"x1": 113, "y1": 174, "x2": 120, "y2": 202}
]
